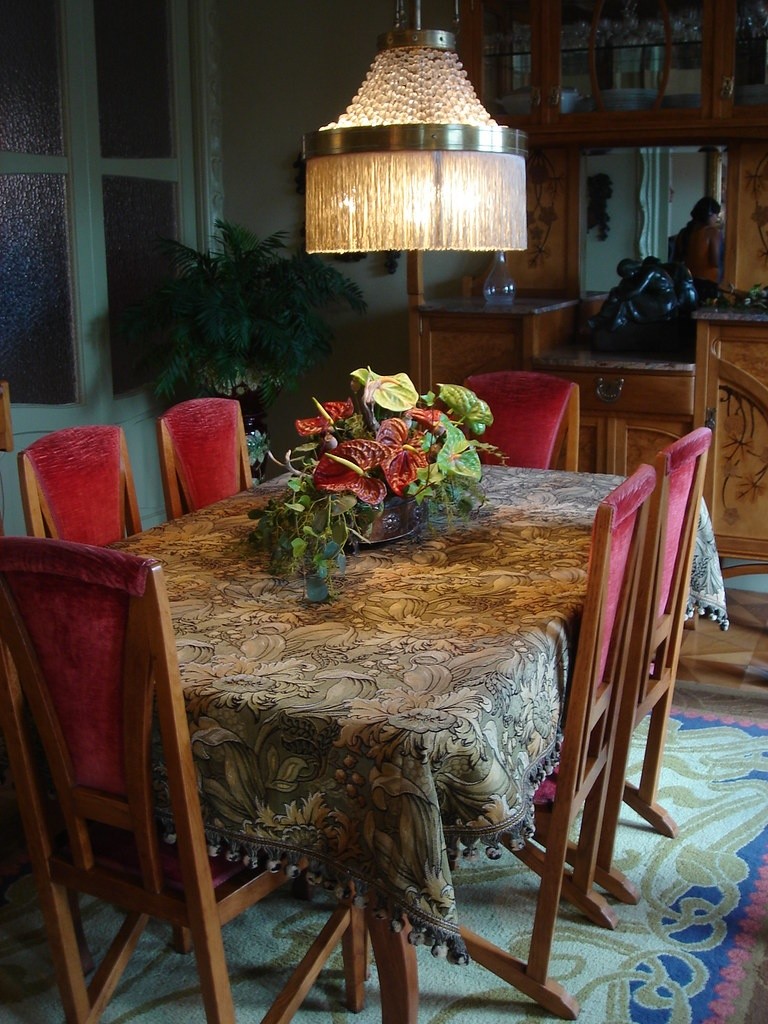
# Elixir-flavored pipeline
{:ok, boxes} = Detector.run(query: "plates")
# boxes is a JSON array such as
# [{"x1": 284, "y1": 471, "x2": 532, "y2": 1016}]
[{"x1": 595, "y1": 83, "x2": 768, "y2": 111}]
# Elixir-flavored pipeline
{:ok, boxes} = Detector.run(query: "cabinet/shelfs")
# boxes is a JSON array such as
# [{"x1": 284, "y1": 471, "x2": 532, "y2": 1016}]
[{"x1": 405, "y1": 0, "x2": 768, "y2": 568}]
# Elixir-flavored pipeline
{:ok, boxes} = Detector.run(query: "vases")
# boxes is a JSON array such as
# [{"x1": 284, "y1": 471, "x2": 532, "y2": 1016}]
[{"x1": 342, "y1": 496, "x2": 427, "y2": 545}]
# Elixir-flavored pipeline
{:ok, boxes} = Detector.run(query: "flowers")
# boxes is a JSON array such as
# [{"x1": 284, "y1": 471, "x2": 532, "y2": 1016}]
[{"x1": 253, "y1": 367, "x2": 507, "y2": 601}]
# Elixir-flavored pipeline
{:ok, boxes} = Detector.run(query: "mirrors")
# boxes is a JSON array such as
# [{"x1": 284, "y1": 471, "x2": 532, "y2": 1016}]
[{"x1": 565, "y1": 137, "x2": 739, "y2": 308}]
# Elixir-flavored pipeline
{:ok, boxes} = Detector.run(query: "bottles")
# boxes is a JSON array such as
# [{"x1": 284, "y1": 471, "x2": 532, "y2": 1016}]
[{"x1": 482, "y1": 251, "x2": 518, "y2": 304}]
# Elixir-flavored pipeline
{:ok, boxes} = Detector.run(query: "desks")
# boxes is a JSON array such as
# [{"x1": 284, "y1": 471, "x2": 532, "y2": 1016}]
[{"x1": 103, "y1": 467, "x2": 730, "y2": 1024}]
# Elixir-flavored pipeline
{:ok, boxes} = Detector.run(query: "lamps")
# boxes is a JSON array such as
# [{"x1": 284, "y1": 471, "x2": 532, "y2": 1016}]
[{"x1": 300, "y1": 0, "x2": 526, "y2": 255}]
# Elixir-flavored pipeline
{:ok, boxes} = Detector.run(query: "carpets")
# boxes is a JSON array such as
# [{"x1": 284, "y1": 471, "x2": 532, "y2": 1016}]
[{"x1": 0, "y1": 681, "x2": 768, "y2": 1024}]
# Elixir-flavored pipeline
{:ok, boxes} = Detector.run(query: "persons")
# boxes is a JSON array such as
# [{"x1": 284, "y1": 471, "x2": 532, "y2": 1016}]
[
  {"x1": 644, "y1": 256, "x2": 698, "y2": 305},
  {"x1": 668, "y1": 197, "x2": 724, "y2": 283},
  {"x1": 599, "y1": 258, "x2": 676, "y2": 326}
]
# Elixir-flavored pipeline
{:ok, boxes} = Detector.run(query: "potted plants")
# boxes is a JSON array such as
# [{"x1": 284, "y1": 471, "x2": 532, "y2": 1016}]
[{"x1": 122, "y1": 222, "x2": 368, "y2": 484}]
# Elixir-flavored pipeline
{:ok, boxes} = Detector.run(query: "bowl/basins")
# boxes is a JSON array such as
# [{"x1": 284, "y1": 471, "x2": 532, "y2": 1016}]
[{"x1": 502, "y1": 92, "x2": 581, "y2": 115}]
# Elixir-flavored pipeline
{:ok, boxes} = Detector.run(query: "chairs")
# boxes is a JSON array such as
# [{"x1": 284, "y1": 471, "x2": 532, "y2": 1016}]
[
  {"x1": 454, "y1": 372, "x2": 582, "y2": 474},
  {"x1": 566, "y1": 428, "x2": 713, "y2": 898},
  {"x1": 430, "y1": 463, "x2": 660, "y2": 1019},
  {"x1": 1, "y1": 535, "x2": 368, "y2": 1024},
  {"x1": 17, "y1": 424, "x2": 143, "y2": 544},
  {"x1": 157, "y1": 396, "x2": 256, "y2": 526}
]
